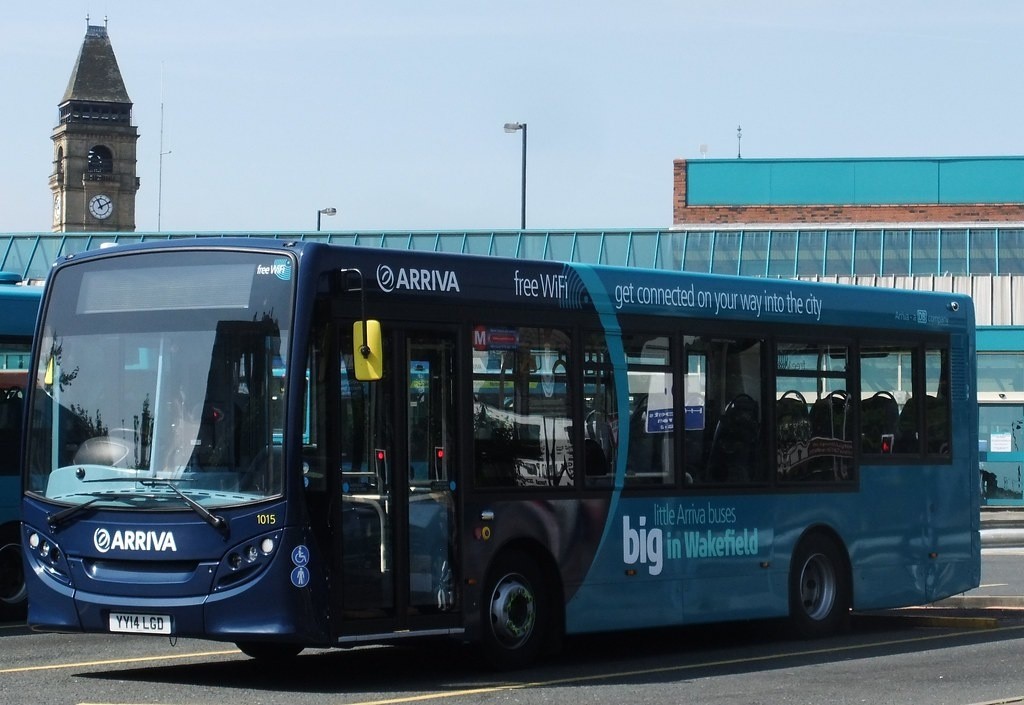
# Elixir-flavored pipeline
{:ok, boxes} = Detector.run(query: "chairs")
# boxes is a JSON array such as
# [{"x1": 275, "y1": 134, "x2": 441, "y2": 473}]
[{"x1": 630, "y1": 390, "x2": 950, "y2": 484}]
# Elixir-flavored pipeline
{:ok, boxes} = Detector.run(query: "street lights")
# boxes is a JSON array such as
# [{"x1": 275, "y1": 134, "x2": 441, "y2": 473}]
[
  {"x1": 504, "y1": 122, "x2": 527, "y2": 229},
  {"x1": 317, "y1": 207, "x2": 337, "y2": 231}
]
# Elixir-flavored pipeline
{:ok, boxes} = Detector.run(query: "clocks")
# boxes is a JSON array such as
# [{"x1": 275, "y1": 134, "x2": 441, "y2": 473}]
[{"x1": 88, "y1": 193, "x2": 114, "y2": 220}]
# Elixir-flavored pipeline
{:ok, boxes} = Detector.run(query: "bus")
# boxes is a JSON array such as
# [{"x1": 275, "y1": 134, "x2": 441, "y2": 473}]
[
  {"x1": 0, "y1": 271, "x2": 88, "y2": 621},
  {"x1": 2, "y1": 239, "x2": 981, "y2": 672}
]
